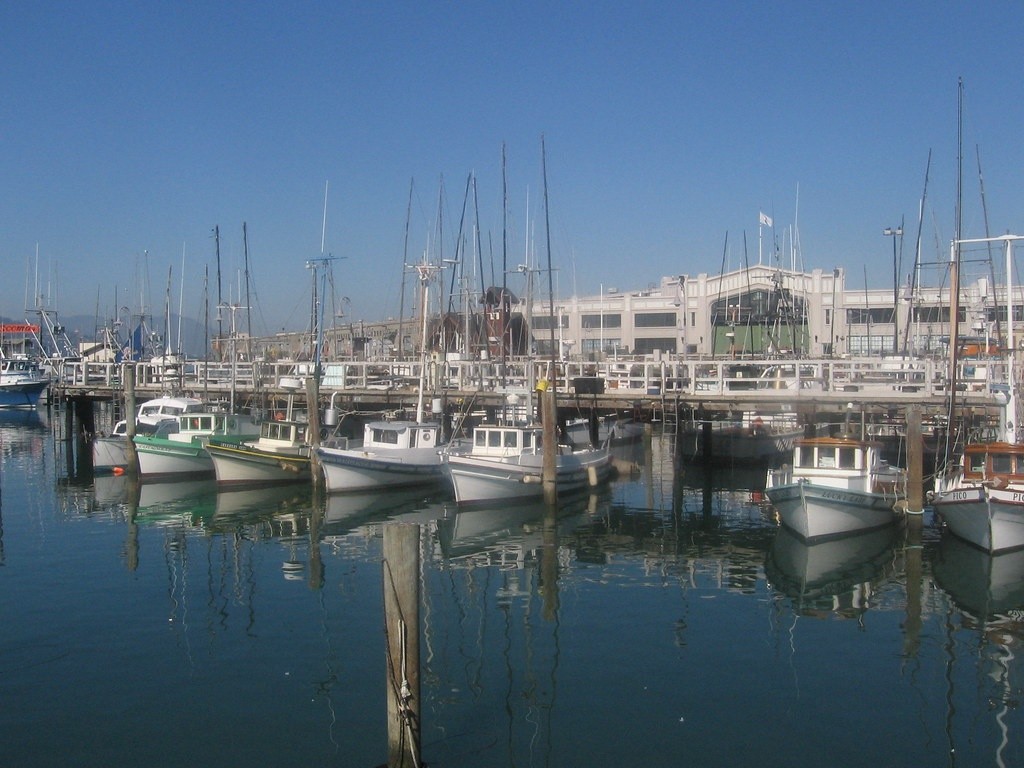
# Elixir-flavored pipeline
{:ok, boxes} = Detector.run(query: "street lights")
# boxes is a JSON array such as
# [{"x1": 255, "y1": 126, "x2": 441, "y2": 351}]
[{"x1": 883, "y1": 227, "x2": 903, "y2": 354}]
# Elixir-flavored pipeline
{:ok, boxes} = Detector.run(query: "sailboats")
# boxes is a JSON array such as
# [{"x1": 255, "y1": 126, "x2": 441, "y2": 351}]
[
  {"x1": 925, "y1": 76, "x2": 1023, "y2": 557},
  {"x1": 0, "y1": 72, "x2": 839, "y2": 556},
  {"x1": 927, "y1": 519, "x2": 1024, "y2": 768}
]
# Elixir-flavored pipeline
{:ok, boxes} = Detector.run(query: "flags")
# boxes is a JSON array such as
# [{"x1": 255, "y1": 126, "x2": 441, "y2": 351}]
[{"x1": 760, "y1": 211, "x2": 773, "y2": 228}]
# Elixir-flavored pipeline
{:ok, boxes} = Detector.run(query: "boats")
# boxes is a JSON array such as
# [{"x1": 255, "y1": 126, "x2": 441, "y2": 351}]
[
  {"x1": 763, "y1": 430, "x2": 907, "y2": 544},
  {"x1": 766, "y1": 518, "x2": 902, "y2": 593}
]
